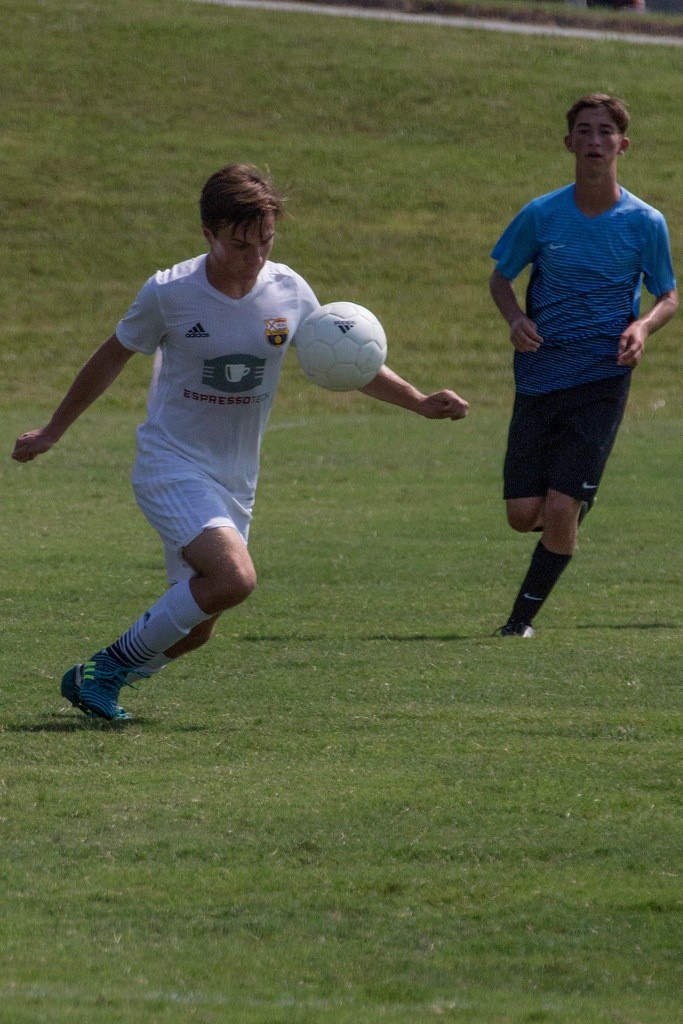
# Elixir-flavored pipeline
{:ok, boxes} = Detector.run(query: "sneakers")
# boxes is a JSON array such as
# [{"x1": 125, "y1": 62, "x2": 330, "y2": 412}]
[
  {"x1": 80, "y1": 650, "x2": 127, "y2": 720},
  {"x1": 61, "y1": 665, "x2": 131, "y2": 726}
]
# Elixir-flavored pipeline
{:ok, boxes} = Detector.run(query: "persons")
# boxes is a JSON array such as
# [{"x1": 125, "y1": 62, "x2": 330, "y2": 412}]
[
  {"x1": 488, "y1": 91, "x2": 679, "y2": 637},
  {"x1": 10, "y1": 163, "x2": 470, "y2": 721}
]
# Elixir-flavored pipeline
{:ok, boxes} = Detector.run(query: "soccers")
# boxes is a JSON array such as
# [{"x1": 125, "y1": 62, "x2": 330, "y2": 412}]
[{"x1": 293, "y1": 299, "x2": 388, "y2": 394}]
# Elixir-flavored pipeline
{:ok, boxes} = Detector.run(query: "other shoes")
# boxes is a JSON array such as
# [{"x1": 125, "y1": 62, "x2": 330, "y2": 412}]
[
  {"x1": 490, "y1": 624, "x2": 534, "y2": 639},
  {"x1": 578, "y1": 497, "x2": 596, "y2": 530}
]
